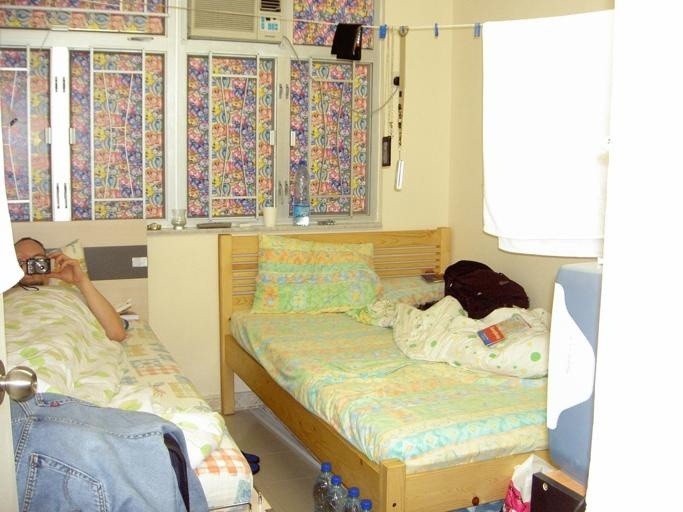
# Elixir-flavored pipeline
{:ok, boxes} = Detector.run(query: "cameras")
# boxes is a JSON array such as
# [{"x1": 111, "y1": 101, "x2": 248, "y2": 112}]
[{"x1": 27, "y1": 257, "x2": 58, "y2": 274}]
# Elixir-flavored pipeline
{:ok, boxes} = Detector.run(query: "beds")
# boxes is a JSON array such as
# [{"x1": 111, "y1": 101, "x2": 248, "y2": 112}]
[
  {"x1": 4, "y1": 218, "x2": 254, "y2": 512},
  {"x1": 218, "y1": 226, "x2": 549, "y2": 512}
]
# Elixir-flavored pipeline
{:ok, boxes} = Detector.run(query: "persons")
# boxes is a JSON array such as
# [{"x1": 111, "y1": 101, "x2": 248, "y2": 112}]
[{"x1": 12, "y1": 236, "x2": 129, "y2": 344}]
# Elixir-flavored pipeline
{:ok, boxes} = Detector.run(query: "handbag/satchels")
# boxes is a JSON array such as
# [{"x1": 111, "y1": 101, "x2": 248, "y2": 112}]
[
  {"x1": 442, "y1": 260, "x2": 528, "y2": 318},
  {"x1": 331, "y1": 23, "x2": 362, "y2": 60}
]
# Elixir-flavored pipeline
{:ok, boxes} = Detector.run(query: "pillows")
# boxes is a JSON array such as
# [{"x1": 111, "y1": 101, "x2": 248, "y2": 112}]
[
  {"x1": 381, "y1": 277, "x2": 444, "y2": 312},
  {"x1": 45, "y1": 238, "x2": 88, "y2": 292},
  {"x1": 252, "y1": 234, "x2": 381, "y2": 314}
]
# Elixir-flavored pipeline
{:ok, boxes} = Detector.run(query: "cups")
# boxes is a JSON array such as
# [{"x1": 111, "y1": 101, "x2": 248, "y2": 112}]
[
  {"x1": 262, "y1": 207, "x2": 277, "y2": 230},
  {"x1": 170, "y1": 208, "x2": 188, "y2": 230}
]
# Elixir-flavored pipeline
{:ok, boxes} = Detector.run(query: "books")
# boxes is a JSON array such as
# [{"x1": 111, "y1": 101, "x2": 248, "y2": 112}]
[
  {"x1": 476, "y1": 313, "x2": 533, "y2": 349},
  {"x1": 114, "y1": 300, "x2": 140, "y2": 322}
]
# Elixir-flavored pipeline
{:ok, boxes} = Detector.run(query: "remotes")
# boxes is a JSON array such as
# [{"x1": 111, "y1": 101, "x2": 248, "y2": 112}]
[{"x1": 197, "y1": 222, "x2": 233, "y2": 228}]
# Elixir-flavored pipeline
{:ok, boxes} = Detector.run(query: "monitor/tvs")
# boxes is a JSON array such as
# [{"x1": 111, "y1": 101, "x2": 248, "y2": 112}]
[{"x1": 545, "y1": 259, "x2": 604, "y2": 484}]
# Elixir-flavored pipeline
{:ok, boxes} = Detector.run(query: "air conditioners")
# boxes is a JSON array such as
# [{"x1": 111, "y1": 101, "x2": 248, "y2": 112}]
[{"x1": 188, "y1": 0, "x2": 283, "y2": 44}]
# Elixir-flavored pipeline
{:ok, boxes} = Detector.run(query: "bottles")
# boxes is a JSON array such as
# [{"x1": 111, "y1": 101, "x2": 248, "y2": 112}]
[
  {"x1": 311, "y1": 461, "x2": 373, "y2": 512},
  {"x1": 292, "y1": 159, "x2": 311, "y2": 226}
]
